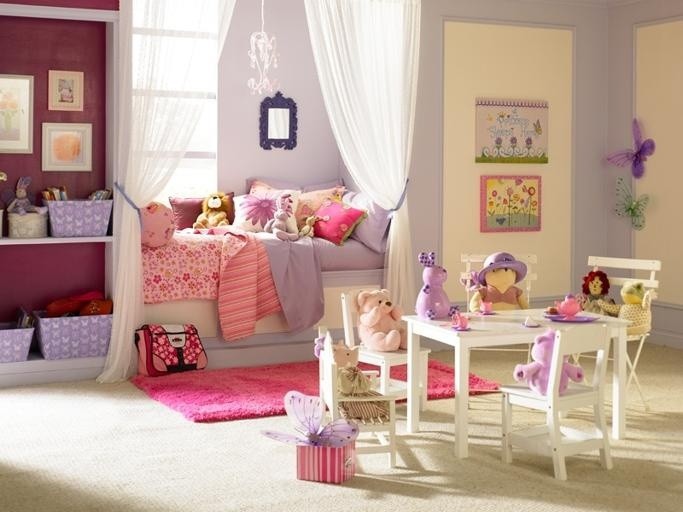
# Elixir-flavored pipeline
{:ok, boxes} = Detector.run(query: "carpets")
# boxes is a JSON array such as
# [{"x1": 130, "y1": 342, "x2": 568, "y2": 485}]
[{"x1": 121, "y1": 353, "x2": 501, "y2": 426}]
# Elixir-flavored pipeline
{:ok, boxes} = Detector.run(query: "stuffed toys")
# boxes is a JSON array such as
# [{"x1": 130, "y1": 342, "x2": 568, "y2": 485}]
[
  {"x1": 543, "y1": 294, "x2": 599, "y2": 323},
  {"x1": 513, "y1": 328, "x2": 585, "y2": 396},
  {"x1": 299, "y1": 214, "x2": 319, "y2": 236},
  {"x1": 596, "y1": 281, "x2": 659, "y2": 336},
  {"x1": 451, "y1": 311, "x2": 471, "y2": 332},
  {"x1": 415, "y1": 252, "x2": 460, "y2": 320},
  {"x1": 469, "y1": 252, "x2": 529, "y2": 313},
  {"x1": 7, "y1": 176, "x2": 48, "y2": 214},
  {"x1": 332, "y1": 339, "x2": 373, "y2": 394},
  {"x1": 263, "y1": 193, "x2": 299, "y2": 242},
  {"x1": 192, "y1": 192, "x2": 230, "y2": 228},
  {"x1": 357, "y1": 288, "x2": 408, "y2": 353}
]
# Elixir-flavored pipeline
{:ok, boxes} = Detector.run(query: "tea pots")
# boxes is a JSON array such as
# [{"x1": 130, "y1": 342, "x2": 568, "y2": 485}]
[{"x1": 553, "y1": 293, "x2": 584, "y2": 319}]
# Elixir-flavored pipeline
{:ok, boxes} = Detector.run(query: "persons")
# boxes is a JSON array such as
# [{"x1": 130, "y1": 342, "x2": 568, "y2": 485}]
[{"x1": 576, "y1": 270, "x2": 615, "y2": 315}]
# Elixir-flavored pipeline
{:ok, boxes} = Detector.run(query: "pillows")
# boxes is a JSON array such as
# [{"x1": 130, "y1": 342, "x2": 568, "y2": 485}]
[{"x1": 136, "y1": 172, "x2": 390, "y2": 255}]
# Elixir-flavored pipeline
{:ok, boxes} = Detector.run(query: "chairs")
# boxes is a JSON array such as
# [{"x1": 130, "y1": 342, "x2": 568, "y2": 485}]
[
  {"x1": 341, "y1": 286, "x2": 431, "y2": 412},
  {"x1": 460, "y1": 254, "x2": 538, "y2": 364},
  {"x1": 571, "y1": 256, "x2": 661, "y2": 411},
  {"x1": 317, "y1": 326, "x2": 400, "y2": 468},
  {"x1": 498, "y1": 324, "x2": 613, "y2": 481}
]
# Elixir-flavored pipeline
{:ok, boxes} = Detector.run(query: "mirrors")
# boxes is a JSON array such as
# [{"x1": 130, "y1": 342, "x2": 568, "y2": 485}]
[{"x1": 257, "y1": 90, "x2": 298, "y2": 153}]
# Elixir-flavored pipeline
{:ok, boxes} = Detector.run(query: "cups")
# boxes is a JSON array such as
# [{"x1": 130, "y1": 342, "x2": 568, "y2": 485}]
[{"x1": 480, "y1": 302, "x2": 492, "y2": 313}]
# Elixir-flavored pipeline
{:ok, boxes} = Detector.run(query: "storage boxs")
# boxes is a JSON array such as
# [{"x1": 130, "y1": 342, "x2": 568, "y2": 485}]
[
  {"x1": 30, "y1": 308, "x2": 115, "y2": 362},
  {"x1": 292, "y1": 442, "x2": 359, "y2": 486},
  {"x1": 0, "y1": 322, "x2": 35, "y2": 363},
  {"x1": 42, "y1": 197, "x2": 113, "y2": 237}
]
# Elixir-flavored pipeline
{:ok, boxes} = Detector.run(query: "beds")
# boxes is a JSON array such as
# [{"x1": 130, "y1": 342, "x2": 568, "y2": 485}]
[{"x1": 135, "y1": 225, "x2": 395, "y2": 347}]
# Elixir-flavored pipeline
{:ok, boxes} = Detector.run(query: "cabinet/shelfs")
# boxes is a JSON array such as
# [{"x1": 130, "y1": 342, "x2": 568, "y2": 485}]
[{"x1": 0, "y1": 1, "x2": 119, "y2": 383}]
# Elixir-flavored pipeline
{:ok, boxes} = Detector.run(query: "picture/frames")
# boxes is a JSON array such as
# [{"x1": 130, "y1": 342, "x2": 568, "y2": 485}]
[
  {"x1": 0, "y1": 74, "x2": 34, "y2": 154},
  {"x1": 477, "y1": 174, "x2": 542, "y2": 233},
  {"x1": 47, "y1": 69, "x2": 84, "y2": 112},
  {"x1": 39, "y1": 119, "x2": 91, "y2": 173}
]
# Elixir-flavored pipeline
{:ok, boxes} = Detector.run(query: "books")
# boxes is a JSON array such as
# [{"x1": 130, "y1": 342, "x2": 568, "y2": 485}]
[
  {"x1": 14, "y1": 304, "x2": 35, "y2": 328},
  {"x1": 42, "y1": 185, "x2": 68, "y2": 201},
  {"x1": 87, "y1": 188, "x2": 112, "y2": 200}
]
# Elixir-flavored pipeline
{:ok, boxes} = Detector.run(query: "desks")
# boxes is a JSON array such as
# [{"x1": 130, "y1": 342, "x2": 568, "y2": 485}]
[{"x1": 402, "y1": 308, "x2": 633, "y2": 459}]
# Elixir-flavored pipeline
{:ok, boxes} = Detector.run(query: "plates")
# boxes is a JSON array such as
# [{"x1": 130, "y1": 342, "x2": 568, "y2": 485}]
[{"x1": 551, "y1": 315, "x2": 600, "y2": 323}]
